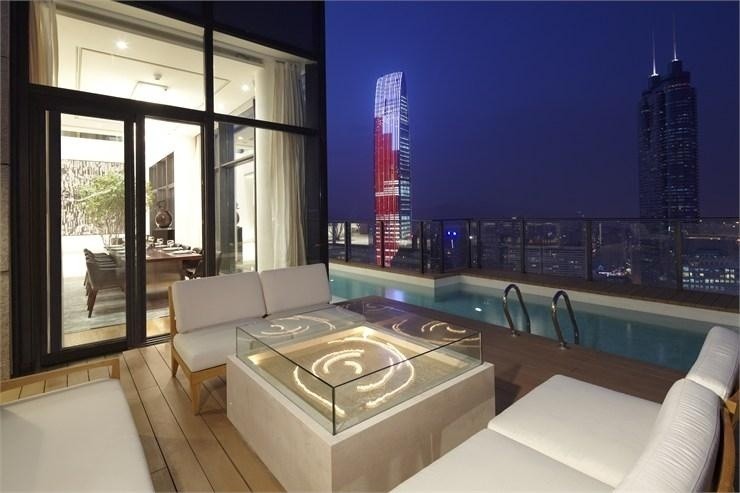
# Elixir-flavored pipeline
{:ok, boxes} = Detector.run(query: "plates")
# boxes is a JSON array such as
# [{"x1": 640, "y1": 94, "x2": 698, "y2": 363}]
[
  {"x1": 173, "y1": 250, "x2": 192, "y2": 254},
  {"x1": 155, "y1": 245, "x2": 167, "y2": 248},
  {"x1": 162, "y1": 247, "x2": 178, "y2": 251}
]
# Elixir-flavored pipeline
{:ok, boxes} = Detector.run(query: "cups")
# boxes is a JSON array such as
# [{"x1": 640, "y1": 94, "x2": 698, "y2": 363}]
[
  {"x1": 156, "y1": 238, "x2": 164, "y2": 245},
  {"x1": 148, "y1": 236, "x2": 155, "y2": 243}
]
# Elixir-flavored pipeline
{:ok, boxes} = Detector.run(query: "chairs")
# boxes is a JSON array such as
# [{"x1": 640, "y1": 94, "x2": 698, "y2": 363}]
[
  {"x1": 725, "y1": 388, "x2": 740, "y2": 433},
  {"x1": 83, "y1": 248, "x2": 124, "y2": 318},
  {"x1": 174, "y1": 241, "x2": 223, "y2": 280}
]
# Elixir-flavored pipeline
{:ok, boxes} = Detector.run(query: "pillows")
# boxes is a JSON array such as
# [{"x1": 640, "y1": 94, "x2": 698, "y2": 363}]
[
  {"x1": 613, "y1": 377, "x2": 721, "y2": 492},
  {"x1": 259, "y1": 263, "x2": 332, "y2": 315},
  {"x1": 685, "y1": 326, "x2": 739, "y2": 407},
  {"x1": 171, "y1": 271, "x2": 267, "y2": 334}
]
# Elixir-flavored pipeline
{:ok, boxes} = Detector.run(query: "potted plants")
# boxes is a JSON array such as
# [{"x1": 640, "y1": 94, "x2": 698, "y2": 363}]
[{"x1": 77, "y1": 169, "x2": 155, "y2": 244}]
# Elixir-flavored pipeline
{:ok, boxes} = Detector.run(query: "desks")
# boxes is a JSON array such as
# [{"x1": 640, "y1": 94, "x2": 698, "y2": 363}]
[{"x1": 104, "y1": 242, "x2": 204, "y2": 292}]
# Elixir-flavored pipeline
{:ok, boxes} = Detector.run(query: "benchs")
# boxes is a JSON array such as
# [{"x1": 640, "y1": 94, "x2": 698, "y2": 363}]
[
  {"x1": 1, "y1": 357, "x2": 157, "y2": 493},
  {"x1": 168, "y1": 286, "x2": 367, "y2": 416},
  {"x1": 386, "y1": 374, "x2": 739, "y2": 493}
]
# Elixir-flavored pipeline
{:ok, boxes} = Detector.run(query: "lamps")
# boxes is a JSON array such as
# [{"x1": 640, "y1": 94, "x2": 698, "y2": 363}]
[{"x1": 129, "y1": 81, "x2": 169, "y2": 104}]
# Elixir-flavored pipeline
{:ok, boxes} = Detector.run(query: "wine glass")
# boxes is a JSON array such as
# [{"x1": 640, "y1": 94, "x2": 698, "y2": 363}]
[{"x1": 167, "y1": 239, "x2": 176, "y2": 248}]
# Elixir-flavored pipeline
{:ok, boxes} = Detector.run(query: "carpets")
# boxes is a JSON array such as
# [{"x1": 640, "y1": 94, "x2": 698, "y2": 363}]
[{"x1": 63, "y1": 268, "x2": 226, "y2": 335}]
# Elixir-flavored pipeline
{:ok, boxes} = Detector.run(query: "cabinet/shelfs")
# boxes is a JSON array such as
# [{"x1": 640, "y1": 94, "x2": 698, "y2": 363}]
[
  {"x1": 152, "y1": 228, "x2": 175, "y2": 245},
  {"x1": 237, "y1": 227, "x2": 242, "y2": 243}
]
